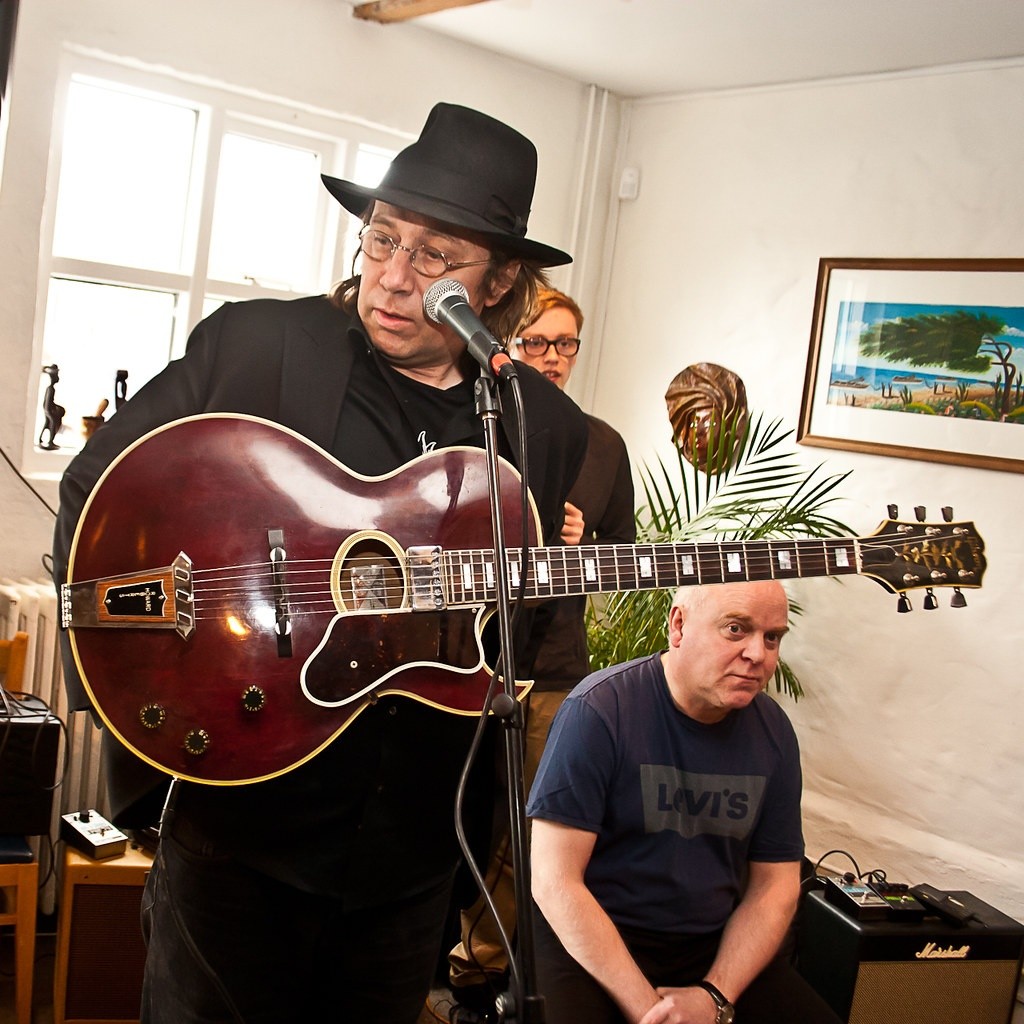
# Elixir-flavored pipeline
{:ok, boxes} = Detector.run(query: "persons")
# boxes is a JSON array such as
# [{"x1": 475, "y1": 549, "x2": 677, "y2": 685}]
[
  {"x1": 425, "y1": 288, "x2": 636, "y2": 1023},
  {"x1": 526, "y1": 580, "x2": 846, "y2": 1024},
  {"x1": 50, "y1": 101, "x2": 589, "y2": 1024}
]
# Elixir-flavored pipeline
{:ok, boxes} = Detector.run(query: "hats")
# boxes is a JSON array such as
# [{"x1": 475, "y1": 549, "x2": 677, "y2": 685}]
[{"x1": 319, "y1": 101, "x2": 573, "y2": 268}]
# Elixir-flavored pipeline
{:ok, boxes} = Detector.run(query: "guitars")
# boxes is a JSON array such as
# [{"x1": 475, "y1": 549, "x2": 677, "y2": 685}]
[{"x1": 63, "y1": 409, "x2": 987, "y2": 790}]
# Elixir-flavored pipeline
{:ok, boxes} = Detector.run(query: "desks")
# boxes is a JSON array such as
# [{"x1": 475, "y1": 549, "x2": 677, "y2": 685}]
[{"x1": 0, "y1": 861, "x2": 40, "y2": 1024}]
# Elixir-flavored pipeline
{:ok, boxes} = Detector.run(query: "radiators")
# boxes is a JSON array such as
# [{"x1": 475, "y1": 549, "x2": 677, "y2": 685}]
[{"x1": 0, "y1": 576, "x2": 131, "y2": 920}]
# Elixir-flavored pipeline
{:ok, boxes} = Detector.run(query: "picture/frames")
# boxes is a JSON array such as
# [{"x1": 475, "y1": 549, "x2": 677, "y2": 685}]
[{"x1": 795, "y1": 258, "x2": 1024, "y2": 475}]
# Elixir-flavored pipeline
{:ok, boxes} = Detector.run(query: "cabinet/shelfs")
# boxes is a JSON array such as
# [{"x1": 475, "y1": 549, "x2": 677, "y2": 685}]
[{"x1": 51, "y1": 839, "x2": 156, "y2": 1024}]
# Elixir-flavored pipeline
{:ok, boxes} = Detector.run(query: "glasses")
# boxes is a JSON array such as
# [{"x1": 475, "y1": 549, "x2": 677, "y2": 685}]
[
  {"x1": 358, "y1": 224, "x2": 495, "y2": 278},
  {"x1": 516, "y1": 335, "x2": 581, "y2": 358}
]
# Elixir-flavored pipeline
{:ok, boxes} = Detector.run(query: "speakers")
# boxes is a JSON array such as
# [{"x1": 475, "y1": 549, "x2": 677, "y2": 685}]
[{"x1": 732, "y1": 889, "x2": 1024, "y2": 1024}]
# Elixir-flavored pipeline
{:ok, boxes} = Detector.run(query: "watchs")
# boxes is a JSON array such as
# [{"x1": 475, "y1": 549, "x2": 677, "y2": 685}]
[{"x1": 697, "y1": 980, "x2": 736, "y2": 1024}]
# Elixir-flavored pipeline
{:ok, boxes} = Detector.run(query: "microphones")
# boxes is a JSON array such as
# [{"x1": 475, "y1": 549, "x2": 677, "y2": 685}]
[{"x1": 422, "y1": 279, "x2": 518, "y2": 381}]
